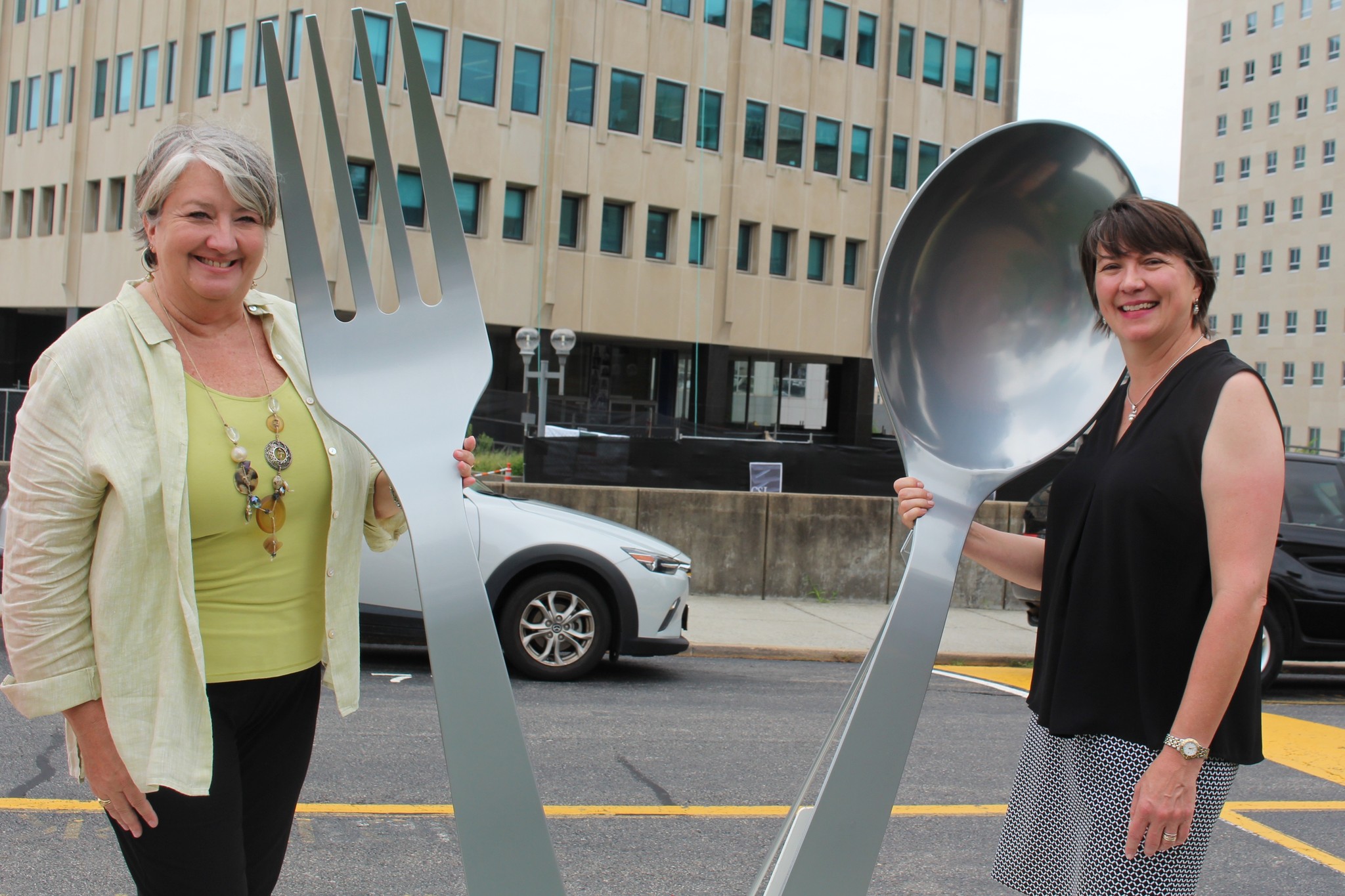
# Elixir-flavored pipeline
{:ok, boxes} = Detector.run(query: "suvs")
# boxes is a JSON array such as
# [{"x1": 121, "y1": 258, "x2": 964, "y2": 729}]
[{"x1": 1015, "y1": 443, "x2": 1345, "y2": 689}]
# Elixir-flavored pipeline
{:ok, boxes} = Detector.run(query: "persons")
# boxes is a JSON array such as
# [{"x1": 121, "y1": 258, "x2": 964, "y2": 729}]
[
  {"x1": 0, "y1": 125, "x2": 475, "y2": 896},
  {"x1": 893, "y1": 198, "x2": 1284, "y2": 896}
]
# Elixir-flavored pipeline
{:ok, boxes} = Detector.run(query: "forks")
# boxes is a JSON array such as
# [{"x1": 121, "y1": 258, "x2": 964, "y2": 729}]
[{"x1": 258, "y1": 2, "x2": 565, "y2": 895}]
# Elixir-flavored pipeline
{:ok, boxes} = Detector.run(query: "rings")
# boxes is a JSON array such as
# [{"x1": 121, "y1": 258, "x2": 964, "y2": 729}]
[
  {"x1": 1162, "y1": 832, "x2": 1178, "y2": 841},
  {"x1": 96, "y1": 797, "x2": 112, "y2": 806},
  {"x1": 471, "y1": 463, "x2": 474, "y2": 468}
]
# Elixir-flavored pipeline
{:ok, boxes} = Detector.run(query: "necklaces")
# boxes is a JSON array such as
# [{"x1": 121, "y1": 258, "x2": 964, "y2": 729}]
[
  {"x1": 150, "y1": 275, "x2": 294, "y2": 561},
  {"x1": 1127, "y1": 333, "x2": 1205, "y2": 422}
]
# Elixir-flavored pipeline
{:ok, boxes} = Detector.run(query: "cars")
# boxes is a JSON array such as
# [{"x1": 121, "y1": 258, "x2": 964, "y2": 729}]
[{"x1": 359, "y1": 451, "x2": 695, "y2": 684}]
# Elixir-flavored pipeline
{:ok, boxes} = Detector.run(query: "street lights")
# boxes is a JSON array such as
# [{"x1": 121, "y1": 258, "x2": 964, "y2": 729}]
[{"x1": 514, "y1": 328, "x2": 579, "y2": 437}]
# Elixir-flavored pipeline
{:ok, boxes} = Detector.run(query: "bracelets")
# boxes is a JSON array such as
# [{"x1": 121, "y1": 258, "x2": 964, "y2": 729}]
[{"x1": 389, "y1": 482, "x2": 402, "y2": 511}]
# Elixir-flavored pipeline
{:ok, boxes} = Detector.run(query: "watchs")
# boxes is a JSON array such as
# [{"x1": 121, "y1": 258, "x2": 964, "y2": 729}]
[{"x1": 1163, "y1": 733, "x2": 1210, "y2": 761}]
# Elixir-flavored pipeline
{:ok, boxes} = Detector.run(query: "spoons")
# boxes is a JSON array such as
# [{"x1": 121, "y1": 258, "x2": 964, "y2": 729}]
[{"x1": 749, "y1": 121, "x2": 1145, "y2": 896}]
